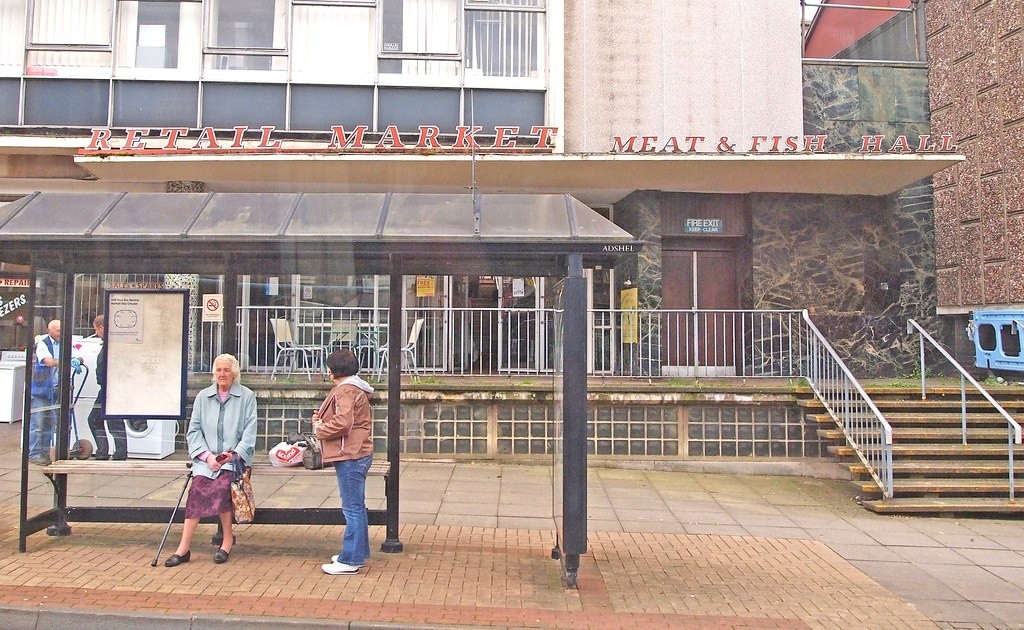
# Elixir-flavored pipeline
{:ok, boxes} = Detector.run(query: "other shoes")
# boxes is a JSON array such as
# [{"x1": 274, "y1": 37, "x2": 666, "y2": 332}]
[
  {"x1": 94, "y1": 456, "x2": 109, "y2": 460},
  {"x1": 30, "y1": 452, "x2": 52, "y2": 466},
  {"x1": 214, "y1": 539, "x2": 232, "y2": 564},
  {"x1": 321, "y1": 561, "x2": 359, "y2": 575},
  {"x1": 165, "y1": 550, "x2": 190, "y2": 567},
  {"x1": 112, "y1": 456, "x2": 126, "y2": 460},
  {"x1": 331, "y1": 555, "x2": 366, "y2": 568}
]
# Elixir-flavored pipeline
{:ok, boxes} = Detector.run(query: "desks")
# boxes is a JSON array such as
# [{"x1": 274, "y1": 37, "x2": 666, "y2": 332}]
[
  {"x1": 318, "y1": 330, "x2": 385, "y2": 381},
  {"x1": 297, "y1": 323, "x2": 390, "y2": 331}
]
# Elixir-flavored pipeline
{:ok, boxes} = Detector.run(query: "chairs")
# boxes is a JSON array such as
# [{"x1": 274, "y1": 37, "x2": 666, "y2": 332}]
[
  {"x1": 356, "y1": 318, "x2": 425, "y2": 383},
  {"x1": 270, "y1": 318, "x2": 329, "y2": 382},
  {"x1": 331, "y1": 319, "x2": 359, "y2": 345}
]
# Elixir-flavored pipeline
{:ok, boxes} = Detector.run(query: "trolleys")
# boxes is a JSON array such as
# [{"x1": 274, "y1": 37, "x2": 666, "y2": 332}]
[{"x1": 50, "y1": 363, "x2": 95, "y2": 461}]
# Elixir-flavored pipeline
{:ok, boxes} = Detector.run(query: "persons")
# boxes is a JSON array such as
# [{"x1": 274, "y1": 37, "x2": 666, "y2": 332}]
[
  {"x1": 88, "y1": 314, "x2": 127, "y2": 460},
  {"x1": 164, "y1": 354, "x2": 257, "y2": 568},
  {"x1": 312, "y1": 349, "x2": 375, "y2": 574},
  {"x1": 29, "y1": 319, "x2": 84, "y2": 466}
]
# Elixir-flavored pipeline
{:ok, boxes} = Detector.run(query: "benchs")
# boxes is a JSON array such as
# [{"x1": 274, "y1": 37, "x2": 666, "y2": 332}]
[{"x1": 41, "y1": 459, "x2": 393, "y2": 499}]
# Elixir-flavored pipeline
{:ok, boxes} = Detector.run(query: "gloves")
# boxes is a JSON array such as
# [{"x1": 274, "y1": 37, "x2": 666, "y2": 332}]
[{"x1": 71, "y1": 358, "x2": 82, "y2": 374}]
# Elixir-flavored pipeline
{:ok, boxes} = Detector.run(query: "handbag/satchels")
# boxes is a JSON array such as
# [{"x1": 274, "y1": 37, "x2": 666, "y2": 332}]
[
  {"x1": 302, "y1": 432, "x2": 336, "y2": 470},
  {"x1": 231, "y1": 452, "x2": 255, "y2": 524},
  {"x1": 269, "y1": 441, "x2": 308, "y2": 468}
]
